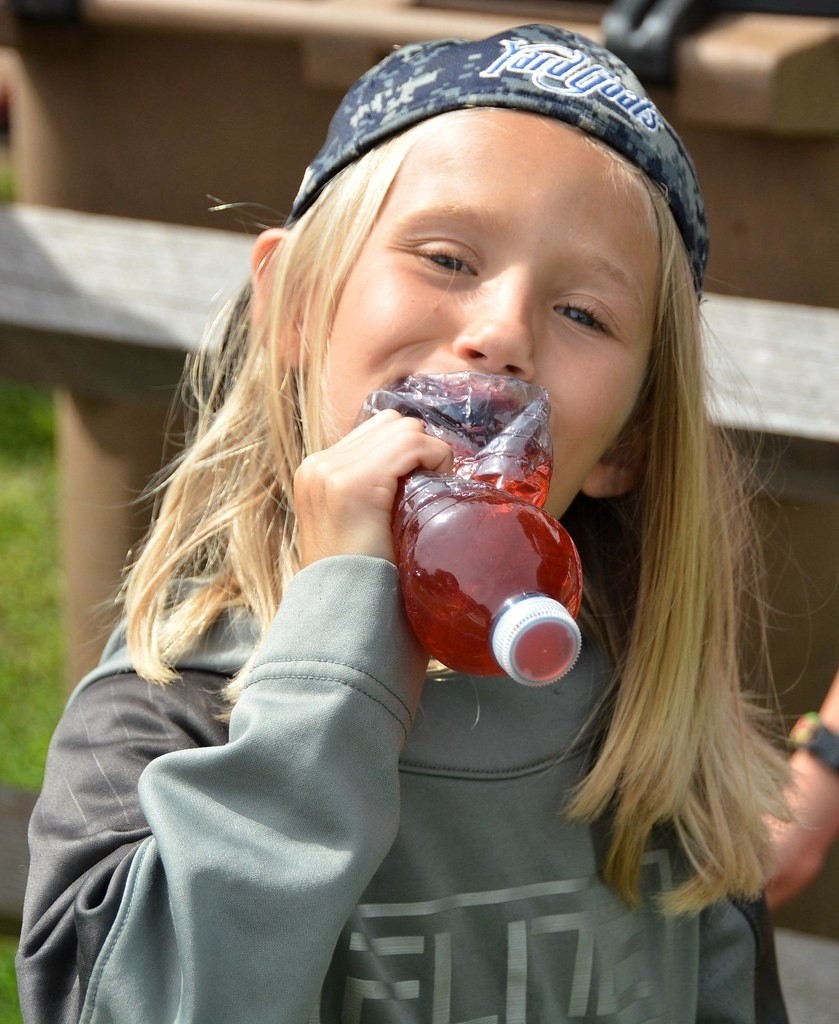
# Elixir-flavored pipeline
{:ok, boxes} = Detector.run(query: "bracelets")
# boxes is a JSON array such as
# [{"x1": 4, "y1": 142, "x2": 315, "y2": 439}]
[{"x1": 785, "y1": 711, "x2": 839, "y2": 775}]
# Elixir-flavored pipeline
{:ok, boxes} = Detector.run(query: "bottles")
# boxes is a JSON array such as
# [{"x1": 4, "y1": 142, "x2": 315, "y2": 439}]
[{"x1": 355, "y1": 372, "x2": 584, "y2": 688}]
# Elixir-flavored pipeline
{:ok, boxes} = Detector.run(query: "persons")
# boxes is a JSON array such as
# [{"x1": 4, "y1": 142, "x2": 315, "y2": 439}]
[{"x1": 1, "y1": 25, "x2": 839, "y2": 1024}]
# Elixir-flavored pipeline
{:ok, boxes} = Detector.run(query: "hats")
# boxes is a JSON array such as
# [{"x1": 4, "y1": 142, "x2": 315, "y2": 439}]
[{"x1": 289, "y1": 24, "x2": 711, "y2": 304}]
